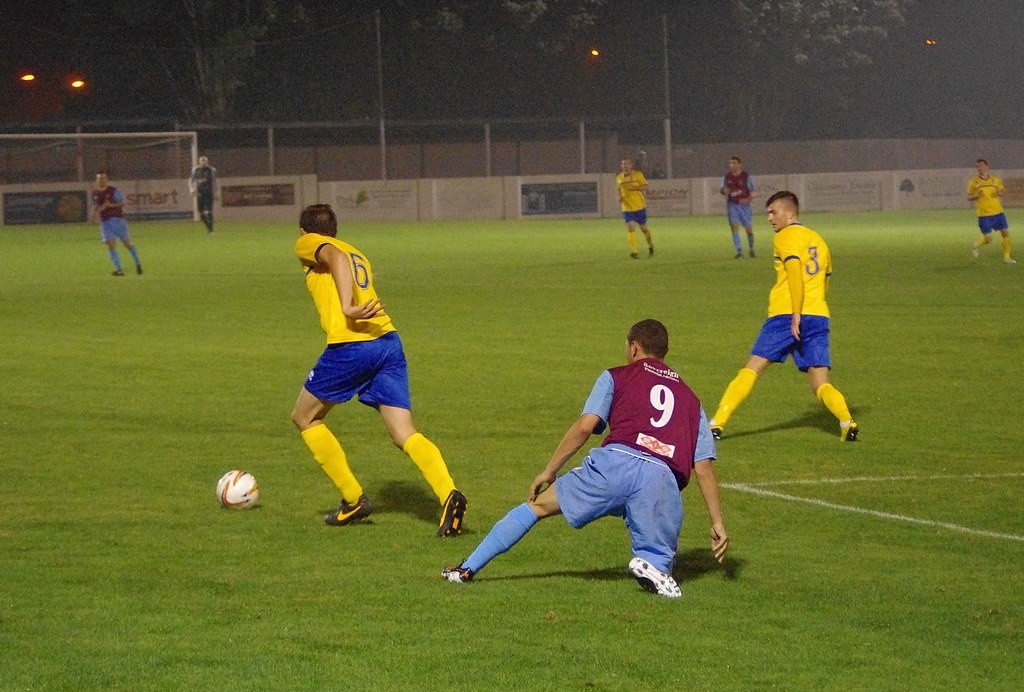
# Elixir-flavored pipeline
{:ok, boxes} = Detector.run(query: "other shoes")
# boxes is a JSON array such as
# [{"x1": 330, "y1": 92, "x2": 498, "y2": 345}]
[
  {"x1": 734, "y1": 253, "x2": 743, "y2": 258},
  {"x1": 972, "y1": 242, "x2": 980, "y2": 258},
  {"x1": 111, "y1": 270, "x2": 124, "y2": 276},
  {"x1": 749, "y1": 249, "x2": 755, "y2": 257},
  {"x1": 648, "y1": 244, "x2": 654, "y2": 256},
  {"x1": 631, "y1": 252, "x2": 640, "y2": 259},
  {"x1": 136, "y1": 264, "x2": 143, "y2": 274},
  {"x1": 1004, "y1": 258, "x2": 1016, "y2": 264}
]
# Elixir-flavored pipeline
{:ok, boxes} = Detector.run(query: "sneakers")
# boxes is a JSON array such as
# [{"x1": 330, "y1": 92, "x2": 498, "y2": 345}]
[
  {"x1": 325, "y1": 490, "x2": 373, "y2": 526},
  {"x1": 442, "y1": 559, "x2": 474, "y2": 584},
  {"x1": 628, "y1": 556, "x2": 683, "y2": 599},
  {"x1": 840, "y1": 419, "x2": 860, "y2": 441},
  {"x1": 708, "y1": 418, "x2": 724, "y2": 440},
  {"x1": 434, "y1": 489, "x2": 468, "y2": 537}
]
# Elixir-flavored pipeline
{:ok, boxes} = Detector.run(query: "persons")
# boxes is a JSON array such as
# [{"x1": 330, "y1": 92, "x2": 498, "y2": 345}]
[
  {"x1": 719, "y1": 156, "x2": 758, "y2": 260},
  {"x1": 615, "y1": 157, "x2": 654, "y2": 258},
  {"x1": 441, "y1": 319, "x2": 729, "y2": 597},
  {"x1": 965, "y1": 159, "x2": 1016, "y2": 263},
  {"x1": 189, "y1": 156, "x2": 219, "y2": 233},
  {"x1": 291, "y1": 203, "x2": 468, "y2": 538},
  {"x1": 709, "y1": 190, "x2": 859, "y2": 442},
  {"x1": 91, "y1": 172, "x2": 143, "y2": 276}
]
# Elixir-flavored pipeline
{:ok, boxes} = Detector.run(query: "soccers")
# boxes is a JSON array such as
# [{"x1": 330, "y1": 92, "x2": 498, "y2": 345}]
[{"x1": 214, "y1": 470, "x2": 260, "y2": 511}]
